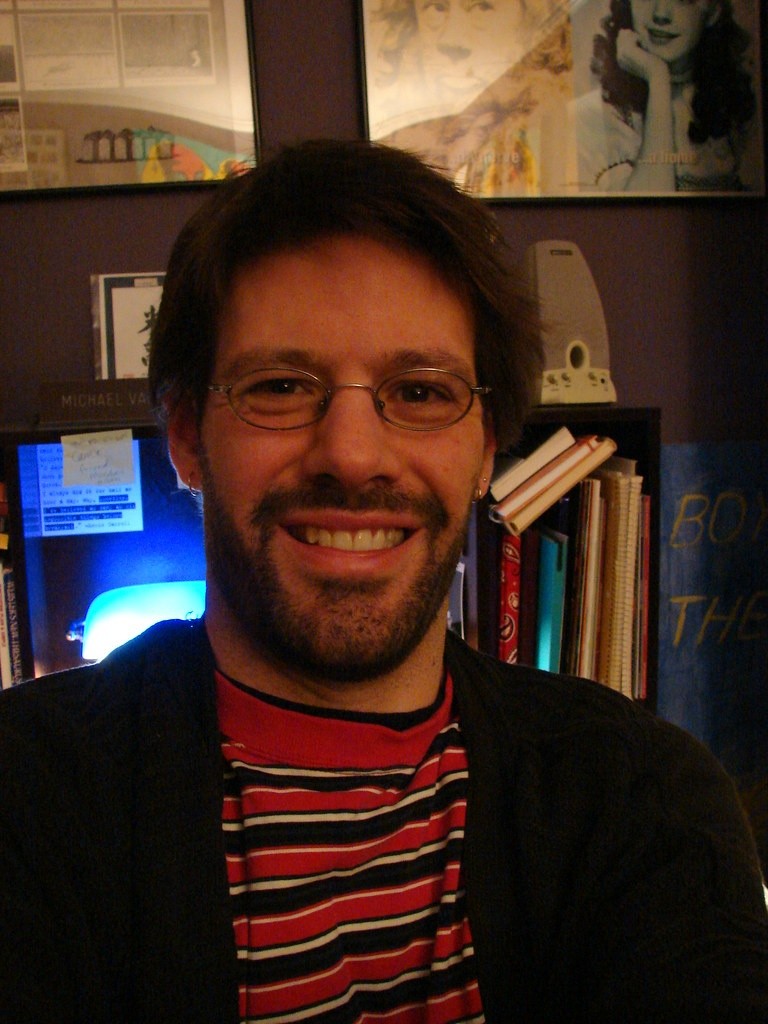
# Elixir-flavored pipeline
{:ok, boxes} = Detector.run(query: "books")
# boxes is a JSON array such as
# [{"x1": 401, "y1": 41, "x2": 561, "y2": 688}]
[
  {"x1": 0, "y1": 479, "x2": 30, "y2": 690},
  {"x1": 487, "y1": 424, "x2": 651, "y2": 702}
]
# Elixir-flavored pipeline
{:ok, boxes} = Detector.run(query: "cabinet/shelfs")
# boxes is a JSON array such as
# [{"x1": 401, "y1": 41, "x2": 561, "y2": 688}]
[{"x1": 0, "y1": 403, "x2": 665, "y2": 715}]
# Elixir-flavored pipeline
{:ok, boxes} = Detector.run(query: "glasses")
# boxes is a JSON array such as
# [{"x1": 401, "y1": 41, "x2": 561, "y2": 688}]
[{"x1": 206, "y1": 365, "x2": 493, "y2": 431}]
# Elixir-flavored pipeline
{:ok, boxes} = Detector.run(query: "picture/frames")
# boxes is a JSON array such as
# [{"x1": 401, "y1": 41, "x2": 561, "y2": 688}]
[{"x1": 357, "y1": 0, "x2": 768, "y2": 207}]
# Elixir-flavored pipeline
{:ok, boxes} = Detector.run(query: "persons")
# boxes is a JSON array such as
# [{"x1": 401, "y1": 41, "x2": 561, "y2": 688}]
[
  {"x1": 569, "y1": 0, "x2": 768, "y2": 194},
  {"x1": 0, "y1": 138, "x2": 768, "y2": 1024}
]
